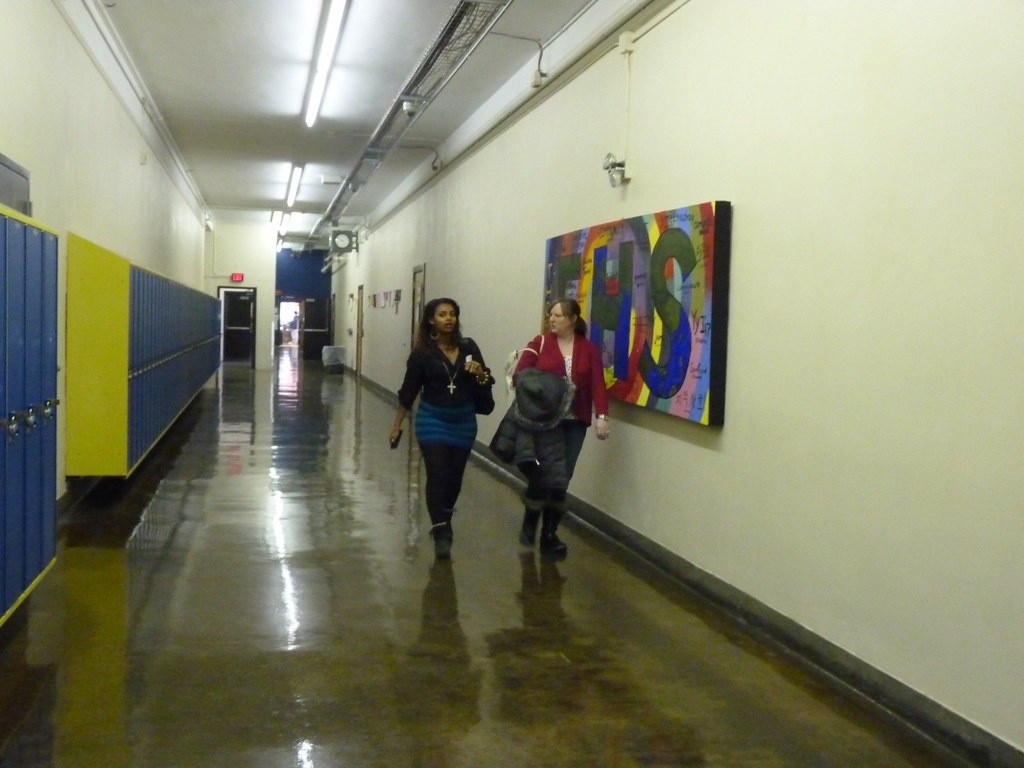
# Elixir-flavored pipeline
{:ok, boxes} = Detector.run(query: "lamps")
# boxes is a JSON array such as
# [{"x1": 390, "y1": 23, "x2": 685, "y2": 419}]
[
  {"x1": 304, "y1": 0, "x2": 352, "y2": 128},
  {"x1": 287, "y1": 162, "x2": 305, "y2": 208},
  {"x1": 603, "y1": 153, "x2": 626, "y2": 186}
]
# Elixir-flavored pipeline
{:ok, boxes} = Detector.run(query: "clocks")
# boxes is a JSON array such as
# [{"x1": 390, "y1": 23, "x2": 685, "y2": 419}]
[{"x1": 335, "y1": 233, "x2": 349, "y2": 249}]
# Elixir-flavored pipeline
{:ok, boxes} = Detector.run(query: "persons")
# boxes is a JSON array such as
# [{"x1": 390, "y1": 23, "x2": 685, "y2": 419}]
[
  {"x1": 389, "y1": 297, "x2": 497, "y2": 562},
  {"x1": 290, "y1": 310, "x2": 299, "y2": 344},
  {"x1": 512, "y1": 299, "x2": 612, "y2": 552}
]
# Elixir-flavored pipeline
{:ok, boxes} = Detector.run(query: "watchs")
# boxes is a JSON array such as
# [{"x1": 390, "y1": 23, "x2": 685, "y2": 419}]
[{"x1": 595, "y1": 414, "x2": 610, "y2": 422}]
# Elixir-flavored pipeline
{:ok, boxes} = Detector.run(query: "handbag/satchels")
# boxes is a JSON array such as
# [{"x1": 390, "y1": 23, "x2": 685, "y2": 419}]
[{"x1": 504, "y1": 334, "x2": 545, "y2": 409}]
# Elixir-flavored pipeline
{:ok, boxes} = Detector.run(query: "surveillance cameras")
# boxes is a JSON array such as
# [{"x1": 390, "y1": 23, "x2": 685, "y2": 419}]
[
  {"x1": 349, "y1": 184, "x2": 359, "y2": 192},
  {"x1": 403, "y1": 100, "x2": 416, "y2": 117}
]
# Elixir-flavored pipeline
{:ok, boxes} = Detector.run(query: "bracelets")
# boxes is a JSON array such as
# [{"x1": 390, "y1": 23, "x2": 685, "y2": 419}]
[{"x1": 474, "y1": 371, "x2": 489, "y2": 385}]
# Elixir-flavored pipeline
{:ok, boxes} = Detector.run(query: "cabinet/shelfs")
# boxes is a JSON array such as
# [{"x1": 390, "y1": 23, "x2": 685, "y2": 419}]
[
  {"x1": 0, "y1": 203, "x2": 57, "y2": 627},
  {"x1": 67, "y1": 231, "x2": 222, "y2": 477}
]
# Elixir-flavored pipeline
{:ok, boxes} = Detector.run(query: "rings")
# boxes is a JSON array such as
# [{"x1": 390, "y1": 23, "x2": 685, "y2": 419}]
[
  {"x1": 473, "y1": 365, "x2": 479, "y2": 369},
  {"x1": 607, "y1": 430, "x2": 610, "y2": 434}
]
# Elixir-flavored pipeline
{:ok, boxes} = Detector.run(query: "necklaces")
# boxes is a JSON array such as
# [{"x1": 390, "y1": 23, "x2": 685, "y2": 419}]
[{"x1": 433, "y1": 340, "x2": 466, "y2": 396}]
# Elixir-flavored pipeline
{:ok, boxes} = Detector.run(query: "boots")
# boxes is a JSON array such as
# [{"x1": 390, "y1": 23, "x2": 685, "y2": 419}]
[
  {"x1": 520, "y1": 508, "x2": 541, "y2": 547},
  {"x1": 444, "y1": 508, "x2": 455, "y2": 539},
  {"x1": 539, "y1": 510, "x2": 567, "y2": 552},
  {"x1": 428, "y1": 522, "x2": 450, "y2": 558}
]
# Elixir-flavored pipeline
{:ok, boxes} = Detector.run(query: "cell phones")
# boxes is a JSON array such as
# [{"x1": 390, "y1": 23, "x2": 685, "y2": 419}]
[{"x1": 391, "y1": 430, "x2": 403, "y2": 448}]
